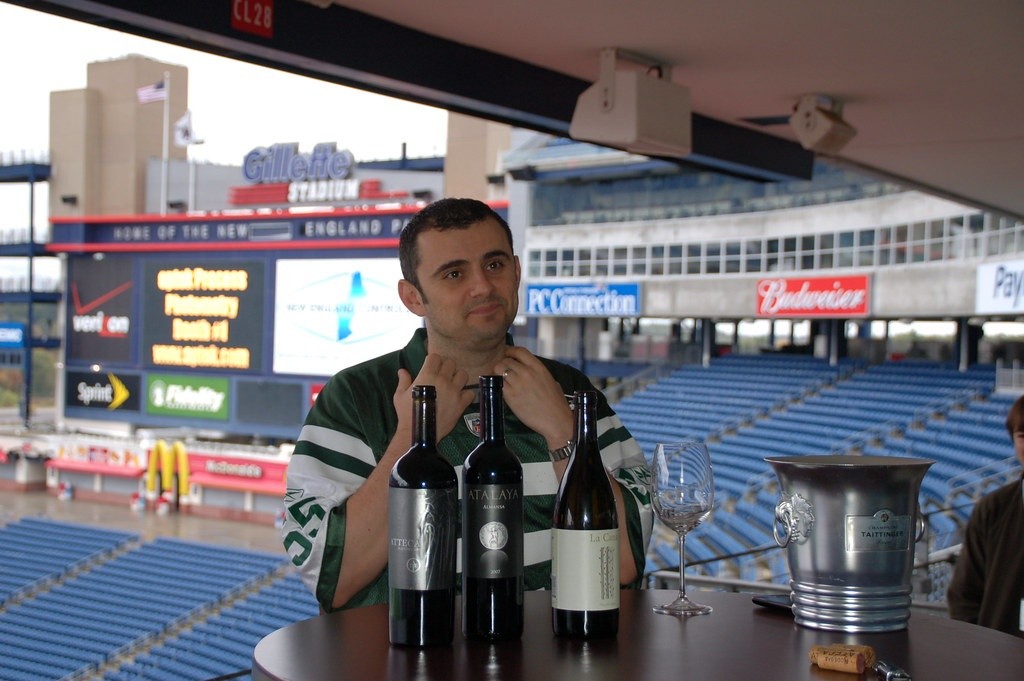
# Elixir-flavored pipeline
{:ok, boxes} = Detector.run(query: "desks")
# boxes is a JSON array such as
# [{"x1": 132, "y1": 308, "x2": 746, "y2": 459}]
[{"x1": 249, "y1": 585, "x2": 1024, "y2": 681}]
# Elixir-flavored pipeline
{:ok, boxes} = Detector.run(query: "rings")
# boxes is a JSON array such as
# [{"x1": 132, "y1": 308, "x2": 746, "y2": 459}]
[{"x1": 501, "y1": 368, "x2": 512, "y2": 380}]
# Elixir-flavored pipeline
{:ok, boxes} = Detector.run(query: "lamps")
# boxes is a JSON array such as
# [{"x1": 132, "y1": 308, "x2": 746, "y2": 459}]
[
  {"x1": 787, "y1": 91, "x2": 861, "y2": 160},
  {"x1": 566, "y1": 43, "x2": 694, "y2": 161}
]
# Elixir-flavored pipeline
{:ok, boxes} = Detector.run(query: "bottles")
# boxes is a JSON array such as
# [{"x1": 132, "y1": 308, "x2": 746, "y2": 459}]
[
  {"x1": 387, "y1": 385, "x2": 458, "y2": 646},
  {"x1": 552, "y1": 391, "x2": 621, "y2": 640},
  {"x1": 462, "y1": 375, "x2": 523, "y2": 638}
]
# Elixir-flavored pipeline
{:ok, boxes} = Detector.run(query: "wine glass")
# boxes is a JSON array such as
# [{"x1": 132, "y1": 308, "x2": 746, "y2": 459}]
[{"x1": 650, "y1": 442, "x2": 715, "y2": 616}]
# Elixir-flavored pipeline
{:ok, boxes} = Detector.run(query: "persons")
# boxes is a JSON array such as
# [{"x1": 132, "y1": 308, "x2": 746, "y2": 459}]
[
  {"x1": 282, "y1": 198, "x2": 655, "y2": 615},
  {"x1": 947, "y1": 394, "x2": 1024, "y2": 640}
]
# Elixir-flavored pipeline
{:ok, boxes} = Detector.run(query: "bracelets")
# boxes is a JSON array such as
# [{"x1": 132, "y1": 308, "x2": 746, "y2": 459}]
[{"x1": 547, "y1": 438, "x2": 575, "y2": 463}]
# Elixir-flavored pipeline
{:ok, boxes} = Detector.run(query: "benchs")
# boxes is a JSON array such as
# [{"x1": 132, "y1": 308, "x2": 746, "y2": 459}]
[{"x1": 0, "y1": 355, "x2": 1024, "y2": 681}]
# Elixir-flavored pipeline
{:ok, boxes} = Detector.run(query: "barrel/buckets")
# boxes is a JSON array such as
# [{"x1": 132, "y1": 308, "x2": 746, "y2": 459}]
[{"x1": 765, "y1": 455, "x2": 936, "y2": 633}]
